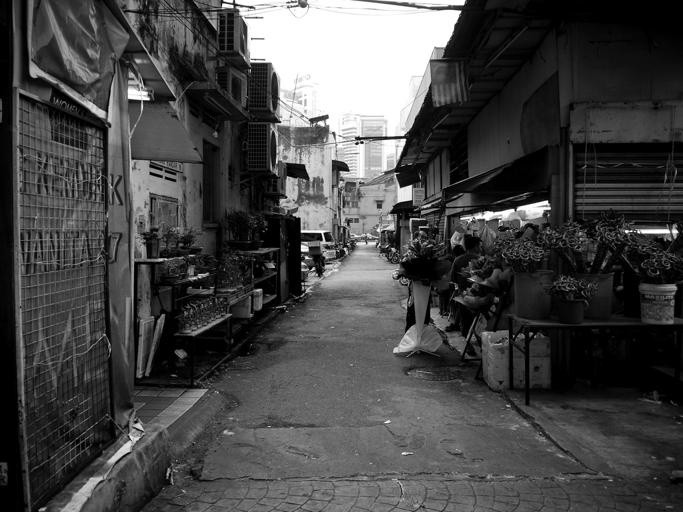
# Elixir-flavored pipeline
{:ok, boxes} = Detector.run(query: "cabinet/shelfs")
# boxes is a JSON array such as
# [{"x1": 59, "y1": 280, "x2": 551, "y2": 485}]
[{"x1": 131, "y1": 245, "x2": 280, "y2": 388}]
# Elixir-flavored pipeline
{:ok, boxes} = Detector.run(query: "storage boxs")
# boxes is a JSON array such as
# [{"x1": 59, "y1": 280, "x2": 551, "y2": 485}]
[{"x1": 480, "y1": 327, "x2": 552, "y2": 392}]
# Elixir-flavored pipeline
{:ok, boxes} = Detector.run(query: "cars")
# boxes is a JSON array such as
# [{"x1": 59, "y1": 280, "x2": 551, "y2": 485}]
[
  {"x1": 301, "y1": 260, "x2": 308, "y2": 278},
  {"x1": 349, "y1": 234, "x2": 360, "y2": 242},
  {"x1": 301, "y1": 242, "x2": 315, "y2": 270},
  {"x1": 358, "y1": 233, "x2": 379, "y2": 242}
]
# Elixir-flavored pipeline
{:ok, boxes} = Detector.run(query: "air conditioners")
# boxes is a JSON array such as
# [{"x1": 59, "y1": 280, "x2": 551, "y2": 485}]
[
  {"x1": 216, "y1": 67, "x2": 247, "y2": 109},
  {"x1": 247, "y1": 60, "x2": 284, "y2": 122},
  {"x1": 261, "y1": 161, "x2": 287, "y2": 200},
  {"x1": 218, "y1": 7, "x2": 251, "y2": 60},
  {"x1": 243, "y1": 122, "x2": 282, "y2": 177}
]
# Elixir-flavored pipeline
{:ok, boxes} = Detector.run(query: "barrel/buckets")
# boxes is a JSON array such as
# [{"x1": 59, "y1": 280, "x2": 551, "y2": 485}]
[{"x1": 639, "y1": 284, "x2": 676, "y2": 326}]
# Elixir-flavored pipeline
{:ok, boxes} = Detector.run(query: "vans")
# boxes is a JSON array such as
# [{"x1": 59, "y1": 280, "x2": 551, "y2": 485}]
[{"x1": 300, "y1": 229, "x2": 337, "y2": 260}]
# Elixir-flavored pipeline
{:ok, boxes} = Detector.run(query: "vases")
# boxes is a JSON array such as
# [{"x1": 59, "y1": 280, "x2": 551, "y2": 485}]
[{"x1": 405, "y1": 282, "x2": 441, "y2": 357}]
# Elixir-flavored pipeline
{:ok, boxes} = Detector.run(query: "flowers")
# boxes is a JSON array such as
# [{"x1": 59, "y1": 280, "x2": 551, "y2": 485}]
[
  {"x1": 501, "y1": 218, "x2": 681, "y2": 326},
  {"x1": 397, "y1": 238, "x2": 451, "y2": 285}
]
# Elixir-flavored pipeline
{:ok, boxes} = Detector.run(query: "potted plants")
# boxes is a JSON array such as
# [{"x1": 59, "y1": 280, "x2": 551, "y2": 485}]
[
  {"x1": 226, "y1": 209, "x2": 268, "y2": 249},
  {"x1": 140, "y1": 226, "x2": 203, "y2": 257}
]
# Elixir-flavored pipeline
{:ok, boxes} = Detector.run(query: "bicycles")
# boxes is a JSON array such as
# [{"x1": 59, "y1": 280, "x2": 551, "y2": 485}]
[{"x1": 383, "y1": 246, "x2": 400, "y2": 265}]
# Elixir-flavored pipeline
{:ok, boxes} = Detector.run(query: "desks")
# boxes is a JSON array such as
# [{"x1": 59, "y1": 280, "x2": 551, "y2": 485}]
[{"x1": 507, "y1": 313, "x2": 683, "y2": 408}]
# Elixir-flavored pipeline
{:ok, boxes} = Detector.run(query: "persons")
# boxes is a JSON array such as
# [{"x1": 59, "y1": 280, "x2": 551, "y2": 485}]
[{"x1": 362, "y1": 232, "x2": 369, "y2": 245}]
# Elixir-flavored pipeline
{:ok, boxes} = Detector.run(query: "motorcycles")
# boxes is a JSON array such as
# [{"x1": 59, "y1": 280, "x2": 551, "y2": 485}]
[{"x1": 307, "y1": 250, "x2": 328, "y2": 278}]
